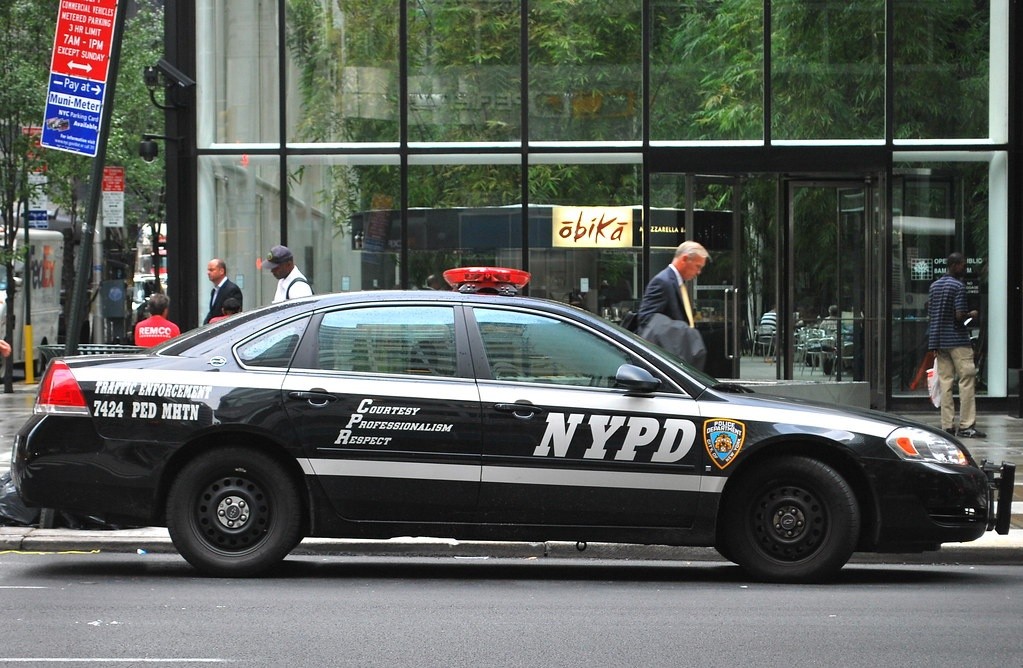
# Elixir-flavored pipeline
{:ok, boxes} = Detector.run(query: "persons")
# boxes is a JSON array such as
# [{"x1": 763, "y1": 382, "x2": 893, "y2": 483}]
[
  {"x1": 0, "y1": 340, "x2": 12, "y2": 357},
  {"x1": 263, "y1": 245, "x2": 312, "y2": 303},
  {"x1": 143, "y1": 281, "x2": 164, "y2": 297},
  {"x1": 637, "y1": 241, "x2": 708, "y2": 370},
  {"x1": 135, "y1": 293, "x2": 180, "y2": 347},
  {"x1": 760, "y1": 307, "x2": 776, "y2": 331},
  {"x1": 203, "y1": 259, "x2": 243, "y2": 324},
  {"x1": 208, "y1": 298, "x2": 239, "y2": 323},
  {"x1": 820, "y1": 305, "x2": 837, "y2": 335},
  {"x1": 928, "y1": 254, "x2": 987, "y2": 438}
]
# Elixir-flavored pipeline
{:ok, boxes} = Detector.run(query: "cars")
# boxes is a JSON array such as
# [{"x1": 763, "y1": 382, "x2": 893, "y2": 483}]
[{"x1": 12, "y1": 267, "x2": 1017, "y2": 585}]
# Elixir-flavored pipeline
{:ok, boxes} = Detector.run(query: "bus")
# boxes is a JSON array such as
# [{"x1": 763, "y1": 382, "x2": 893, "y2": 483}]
[
  {"x1": 0, "y1": 224, "x2": 64, "y2": 378},
  {"x1": 128, "y1": 221, "x2": 169, "y2": 347}
]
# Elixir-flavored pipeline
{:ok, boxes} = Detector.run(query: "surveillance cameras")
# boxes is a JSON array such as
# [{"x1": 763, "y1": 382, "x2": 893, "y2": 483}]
[
  {"x1": 139, "y1": 141, "x2": 158, "y2": 163},
  {"x1": 156, "y1": 57, "x2": 197, "y2": 90}
]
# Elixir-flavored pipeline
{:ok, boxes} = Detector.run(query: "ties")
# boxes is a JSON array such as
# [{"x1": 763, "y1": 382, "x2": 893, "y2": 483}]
[{"x1": 680, "y1": 283, "x2": 694, "y2": 328}]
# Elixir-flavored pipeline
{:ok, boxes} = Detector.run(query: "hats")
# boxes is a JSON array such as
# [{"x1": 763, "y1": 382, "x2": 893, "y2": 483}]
[{"x1": 262, "y1": 245, "x2": 292, "y2": 269}]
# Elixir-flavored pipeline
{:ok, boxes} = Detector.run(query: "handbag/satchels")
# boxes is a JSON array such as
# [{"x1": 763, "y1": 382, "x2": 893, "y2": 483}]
[{"x1": 926, "y1": 356, "x2": 942, "y2": 408}]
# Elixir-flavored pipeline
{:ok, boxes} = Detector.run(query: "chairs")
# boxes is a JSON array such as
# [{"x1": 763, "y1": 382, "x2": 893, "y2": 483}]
[{"x1": 751, "y1": 326, "x2": 853, "y2": 381}]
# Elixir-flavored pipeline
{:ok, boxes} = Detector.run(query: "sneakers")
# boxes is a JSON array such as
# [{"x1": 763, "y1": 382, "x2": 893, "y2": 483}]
[
  {"x1": 946, "y1": 426, "x2": 956, "y2": 437},
  {"x1": 957, "y1": 422, "x2": 986, "y2": 438}
]
